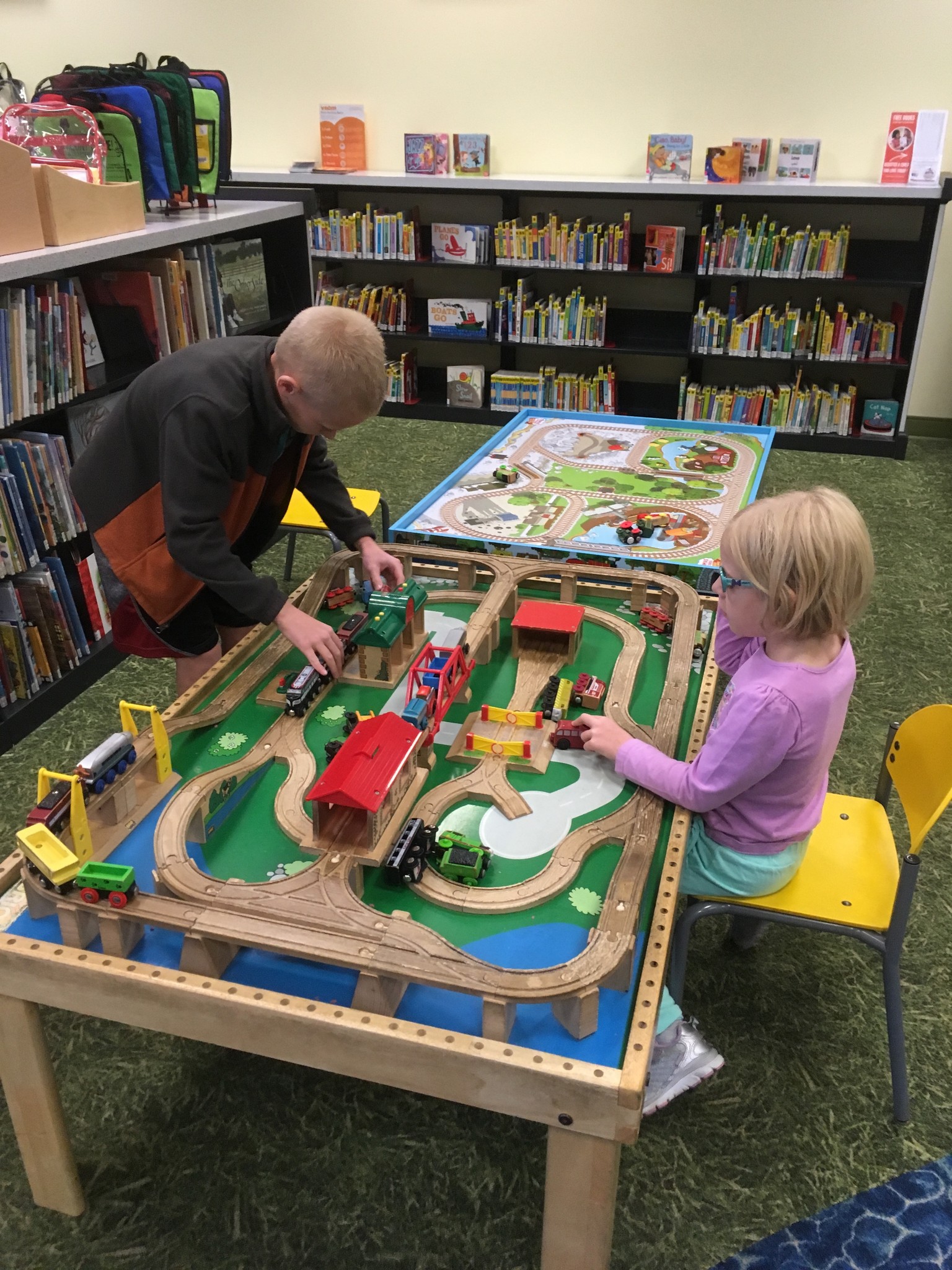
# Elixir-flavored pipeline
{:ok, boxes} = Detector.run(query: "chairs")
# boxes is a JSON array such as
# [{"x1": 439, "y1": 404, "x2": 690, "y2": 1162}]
[{"x1": 666, "y1": 703, "x2": 951, "y2": 1127}]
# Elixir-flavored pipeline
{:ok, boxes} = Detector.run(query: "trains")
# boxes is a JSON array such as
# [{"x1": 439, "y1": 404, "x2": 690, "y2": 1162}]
[
  {"x1": 548, "y1": 718, "x2": 591, "y2": 751},
  {"x1": 540, "y1": 675, "x2": 573, "y2": 723},
  {"x1": 16, "y1": 730, "x2": 138, "y2": 909},
  {"x1": 324, "y1": 741, "x2": 344, "y2": 763},
  {"x1": 284, "y1": 584, "x2": 390, "y2": 718},
  {"x1": 573, "y1": 673, "x2": 606, "y2": 710},
  {"x1": 342, "y1": 709, "x2": 374, "y2": 735},
  {"x1": 616, "y1": 513, "x2": 671, "y2": 545},
  {"x1": 639, "y1": 606, "x2": 674, "y2": 634},
  {"x1": 435, "y1": 830, "x2": 492, "y2": 888},
  {"x1": 383, "y1": 818, "x2": 439, "y2": 888},
  {"x1": 493, "y1": 465, "x2": 520, "y2": 483}
]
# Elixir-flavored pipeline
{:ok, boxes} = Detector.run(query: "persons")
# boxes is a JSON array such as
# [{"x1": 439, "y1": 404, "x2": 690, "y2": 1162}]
[
  {"x1": 581, "y1": 484, "x2": 872, "y2": 1120},
  {"x1": 69, "y1": 303, "x2": 406, "y2": 685}
]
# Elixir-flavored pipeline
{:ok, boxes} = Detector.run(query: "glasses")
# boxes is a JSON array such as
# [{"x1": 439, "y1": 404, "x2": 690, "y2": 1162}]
[{"x1": 718, "y1": 565, "x2": 753, "y2": 593}]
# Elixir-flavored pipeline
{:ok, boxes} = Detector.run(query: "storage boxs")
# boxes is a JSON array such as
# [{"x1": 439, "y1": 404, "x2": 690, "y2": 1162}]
[{"x1": 0, "y1": 138, "x2": 145, "y2": 256}]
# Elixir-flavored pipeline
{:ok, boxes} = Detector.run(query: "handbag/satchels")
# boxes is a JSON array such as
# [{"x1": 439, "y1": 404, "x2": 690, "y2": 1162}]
[
  {"x1": 31, "y1": 52, "x2": 233, "y2": 208},
  {"x1": 0, "y1": 61, "x2": 28, "y2": 119},
  {"x1": 0, "y1": 93, "x2": 107, "y2": 185}
]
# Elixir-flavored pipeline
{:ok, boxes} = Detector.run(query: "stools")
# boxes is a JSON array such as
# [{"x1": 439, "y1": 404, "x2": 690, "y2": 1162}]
[{"x1": 260, "y1": 488, "x2": 390, "y2": 580}]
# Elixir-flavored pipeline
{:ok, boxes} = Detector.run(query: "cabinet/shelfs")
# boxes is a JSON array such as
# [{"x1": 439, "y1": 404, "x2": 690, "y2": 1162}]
[
  {"x1": 218, "y1": 171, "x2": 952, "y2": 458},
  {"x1": 0, "y1": 199, "x2": 315, "y2": 756}
]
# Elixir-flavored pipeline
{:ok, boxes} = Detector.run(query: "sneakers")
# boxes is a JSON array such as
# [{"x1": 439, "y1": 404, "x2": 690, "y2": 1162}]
[{"x1": 642, "y1": 1014, "x2": 727, "y2": 1118}]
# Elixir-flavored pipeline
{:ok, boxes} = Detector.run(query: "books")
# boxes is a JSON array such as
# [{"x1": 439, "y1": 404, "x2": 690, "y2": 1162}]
[
  {"x1": 880, "y1": 110, "x2": 946, "y2": 186},
  {"x1": 0, "y1": 237, "x2": 273, "y2": 424},
  {"x1": 431, "y1": 211, "x2": 631, "y2": 272},
  {"x1": 676, "y1": 367, "x2": 901, "y2": 441},
  {"x1": 402, "y1": 132, "x2": 490, "y2": 176},
  {"x1": 427, "y1": 275, "x2": 606, "y2": 347},
  {"x1": 308, "y1": 203, "x2": 415, "y2": 261},
  {"x1": 691, "y1": 285, "x2": 896, "y2": 361},
  {"x1": 646, "y1": 133, "x2": 692, "y2": 181},
  {"x1": 698, "y1": 204, "x2": 850, "y2": 278},
  {"x1": 706, "y1": 134, "x2": 820, "y2": 183},
  {"x1": 446, "y1": 357, "x2": 615, "y2": 414},
  {"x1": 0, "y1": 431, "x2": 112, "y2": 708},
  {"x1": 317, "y1": 105, "x2": 367, "y2": 174},
  {"x1": 315, "y1": 268, "x2": 422, "y2": 404},
  {"x1": 644, "y1": 225, "x2": 685, "y2": 273}
]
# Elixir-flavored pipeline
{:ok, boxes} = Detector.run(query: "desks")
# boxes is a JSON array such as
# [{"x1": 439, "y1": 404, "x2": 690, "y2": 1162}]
[
  {"x1": 0, "y1": 562, "x2": 721, "y2": 1270},
  {"x1": 387, "y1": 408, "x2": 776, "y2": 594}
]
